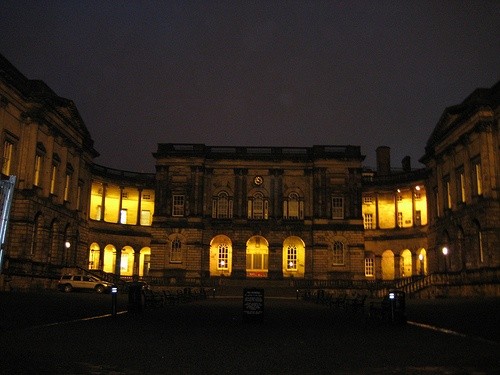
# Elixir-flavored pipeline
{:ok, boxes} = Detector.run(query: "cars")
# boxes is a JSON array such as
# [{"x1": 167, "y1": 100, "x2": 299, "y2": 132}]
[{"x1": 137, "y1": 281, "x2": 153, "y2": 293}]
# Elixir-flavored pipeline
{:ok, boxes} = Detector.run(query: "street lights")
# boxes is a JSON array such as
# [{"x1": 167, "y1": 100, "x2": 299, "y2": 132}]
[
  {"x1": 65, "y1": 241, "x2": 71, "y2": 276},
  {"x1": 442, "y1": 246, "x2": 451, "y2": 300}
]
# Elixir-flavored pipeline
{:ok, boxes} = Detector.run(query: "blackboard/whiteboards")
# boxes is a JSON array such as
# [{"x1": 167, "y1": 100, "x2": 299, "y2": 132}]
[{"x1": 241, "y1": 287, "x2": 265, "y2": 324}]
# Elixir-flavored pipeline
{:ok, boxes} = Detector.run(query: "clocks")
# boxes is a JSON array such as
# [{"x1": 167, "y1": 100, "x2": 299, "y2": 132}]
[{"x1": 254, "y1": 176, "x2": 264, "y2": 186}]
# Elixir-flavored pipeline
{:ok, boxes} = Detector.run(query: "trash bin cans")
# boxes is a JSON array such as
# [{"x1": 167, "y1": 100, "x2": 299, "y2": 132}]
[{"x1": 384, "y1": 290, "x2": 407, "y2": 329}]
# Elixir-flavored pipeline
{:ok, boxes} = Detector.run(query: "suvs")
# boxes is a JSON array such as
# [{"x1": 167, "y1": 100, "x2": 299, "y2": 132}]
[{"x1": 57, "y1": 275, "x2": 114, "y2": 293}]
